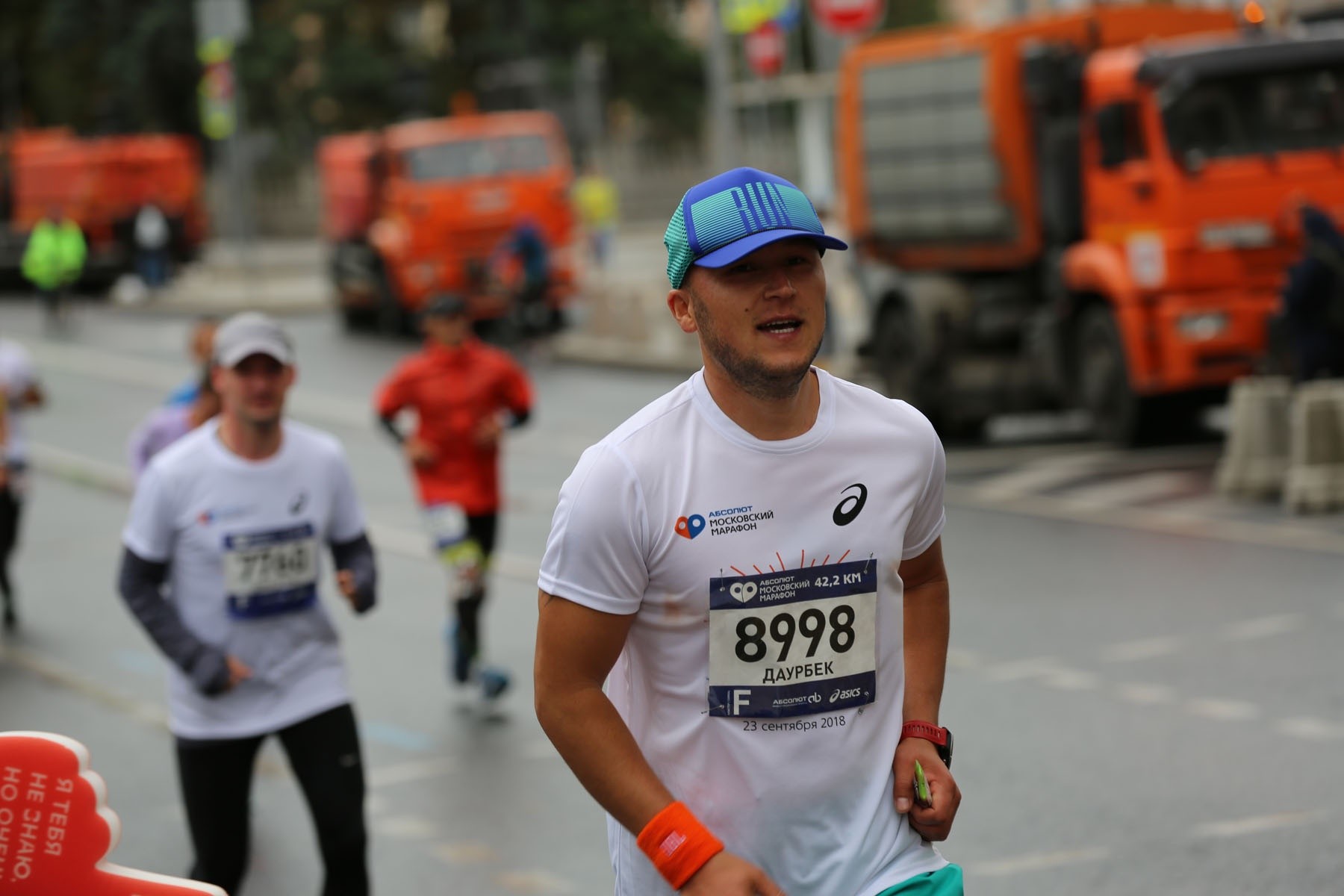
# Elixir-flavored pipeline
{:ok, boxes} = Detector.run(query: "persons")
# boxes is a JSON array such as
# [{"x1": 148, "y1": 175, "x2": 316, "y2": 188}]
[
  {"x1": 536, "y1": 167, "x2": 970, "y2": 896},
  {"x1": 0, "y1": 151, "x2": 622, "y2": 896},
  {"x1": 1207, "y1": 196, "x2": 1344, "y2": 433}
]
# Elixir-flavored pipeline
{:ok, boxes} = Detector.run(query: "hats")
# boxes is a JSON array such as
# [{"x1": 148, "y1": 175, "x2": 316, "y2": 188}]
[
  {"x1": 663, "y1": 167, "x2": 848, "y2": 289},
  {"x1": 210, "y1": 311, "x2": 297, "y2": 369}
]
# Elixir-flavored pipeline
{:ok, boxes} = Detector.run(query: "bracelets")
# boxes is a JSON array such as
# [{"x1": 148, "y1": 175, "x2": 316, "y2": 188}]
[{"x1": 636, "y1": 803, "x2": 727, "y2": 891}]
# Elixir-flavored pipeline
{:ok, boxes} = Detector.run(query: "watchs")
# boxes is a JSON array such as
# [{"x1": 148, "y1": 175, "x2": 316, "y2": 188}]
[{"x1": 902, "y1": 720, "x2": 955, "y2": 771}]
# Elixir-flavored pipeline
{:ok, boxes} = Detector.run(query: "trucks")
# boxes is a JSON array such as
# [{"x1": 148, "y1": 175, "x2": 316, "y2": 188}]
[
  {"x1": 830, "y1": 0, "x2": 1344, "y2": 454},
  {"x1": 0, "y1": 96, "x2": 216, "y2": 301},
  {"x1": 316, "y1": 106, "x2": 583, "y2": 342}
]
